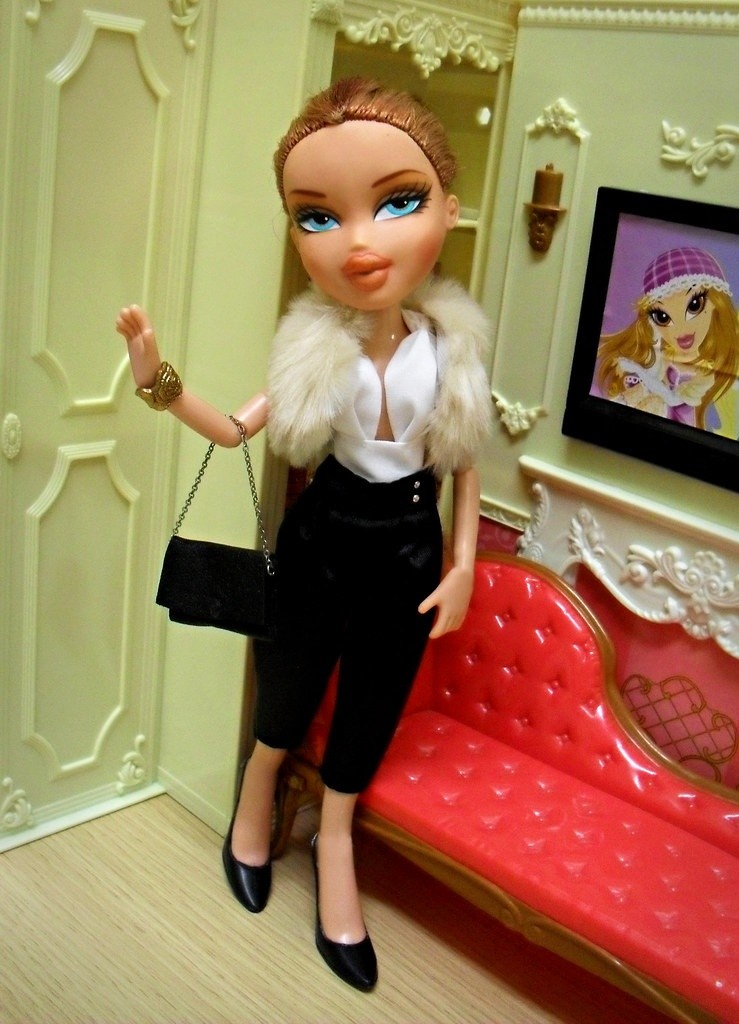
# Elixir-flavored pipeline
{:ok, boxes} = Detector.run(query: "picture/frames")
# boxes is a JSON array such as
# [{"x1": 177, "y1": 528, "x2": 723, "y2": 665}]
[{"x1": 561, "y1": 185, "x2": 739, "y2": 493}]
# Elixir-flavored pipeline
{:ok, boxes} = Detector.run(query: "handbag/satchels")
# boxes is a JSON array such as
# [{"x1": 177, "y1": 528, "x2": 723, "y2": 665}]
[{"x1": 156, "y1": 412, "x2": 293, "y2": 640}]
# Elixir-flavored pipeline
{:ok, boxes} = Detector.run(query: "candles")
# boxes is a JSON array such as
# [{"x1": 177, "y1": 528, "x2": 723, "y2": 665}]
[{"x1": 531, "y1": 161, "x2": 563, "y2": 208}]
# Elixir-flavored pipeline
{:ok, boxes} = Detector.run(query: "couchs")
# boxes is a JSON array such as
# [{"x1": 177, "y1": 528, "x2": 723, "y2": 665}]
[{"x1": 273, "y1": 549, "x2": 739, "y2": 1024}]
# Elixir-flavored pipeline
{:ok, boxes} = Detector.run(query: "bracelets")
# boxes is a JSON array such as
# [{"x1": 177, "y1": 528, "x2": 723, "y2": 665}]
[{"x1": 135, "y1": 361, "x2": 182, "y2": 411}]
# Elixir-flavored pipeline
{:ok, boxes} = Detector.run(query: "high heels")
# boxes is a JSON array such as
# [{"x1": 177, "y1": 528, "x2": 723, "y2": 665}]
[
  {"x1": 306, "y1": 829, "x2": 377, "y2": 991},
  {"x1": 221, "y1": 760, "x2": 274, "y2": 914}
]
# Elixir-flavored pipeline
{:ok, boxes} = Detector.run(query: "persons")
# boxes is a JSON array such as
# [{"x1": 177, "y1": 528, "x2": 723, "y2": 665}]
[{"x1": 114, "y1": 74, "x2": 491, "y2": 995}]
